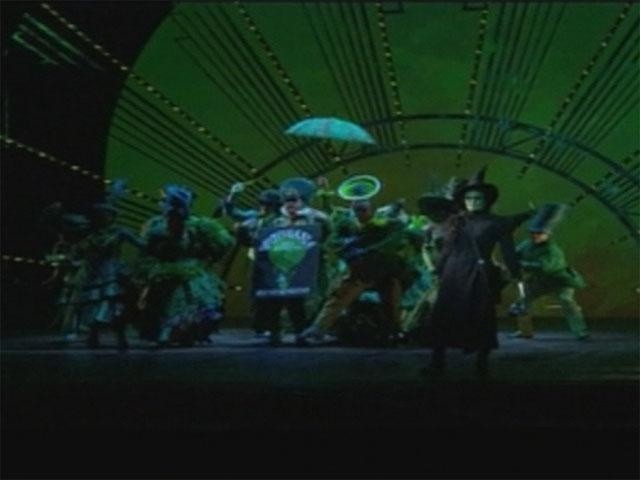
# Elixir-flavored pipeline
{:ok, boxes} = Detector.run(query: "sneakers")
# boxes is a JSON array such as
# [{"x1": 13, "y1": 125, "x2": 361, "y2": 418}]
[
  {"x1": 416, "y1": 347, "x2": 486, "y2": 378},
  {"x1": 509, "y1": 332, "x2": 594, "y2": 341}
]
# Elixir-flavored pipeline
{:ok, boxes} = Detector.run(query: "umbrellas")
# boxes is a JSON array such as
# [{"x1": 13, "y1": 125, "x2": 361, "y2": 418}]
[{"x1": 282, "y1": 117, "x2": 379, "y2": 176}]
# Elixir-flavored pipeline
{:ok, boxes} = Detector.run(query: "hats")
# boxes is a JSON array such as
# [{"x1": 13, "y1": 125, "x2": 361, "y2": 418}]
[
  {"x1": 417, "y1": 166, "x2": 565, "y2": 237},
  {"x1": 91, "y1": 188, "x2": 299, "y2": 225}
]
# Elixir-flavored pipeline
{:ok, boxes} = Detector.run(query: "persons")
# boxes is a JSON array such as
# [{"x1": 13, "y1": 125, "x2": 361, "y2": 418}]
[
  {"x1": 214, "y1": 178, "x2": 590, "y2": 355},
  {"x1": 36, "y1": 186, "x2": 238, "y2": 349}
]
